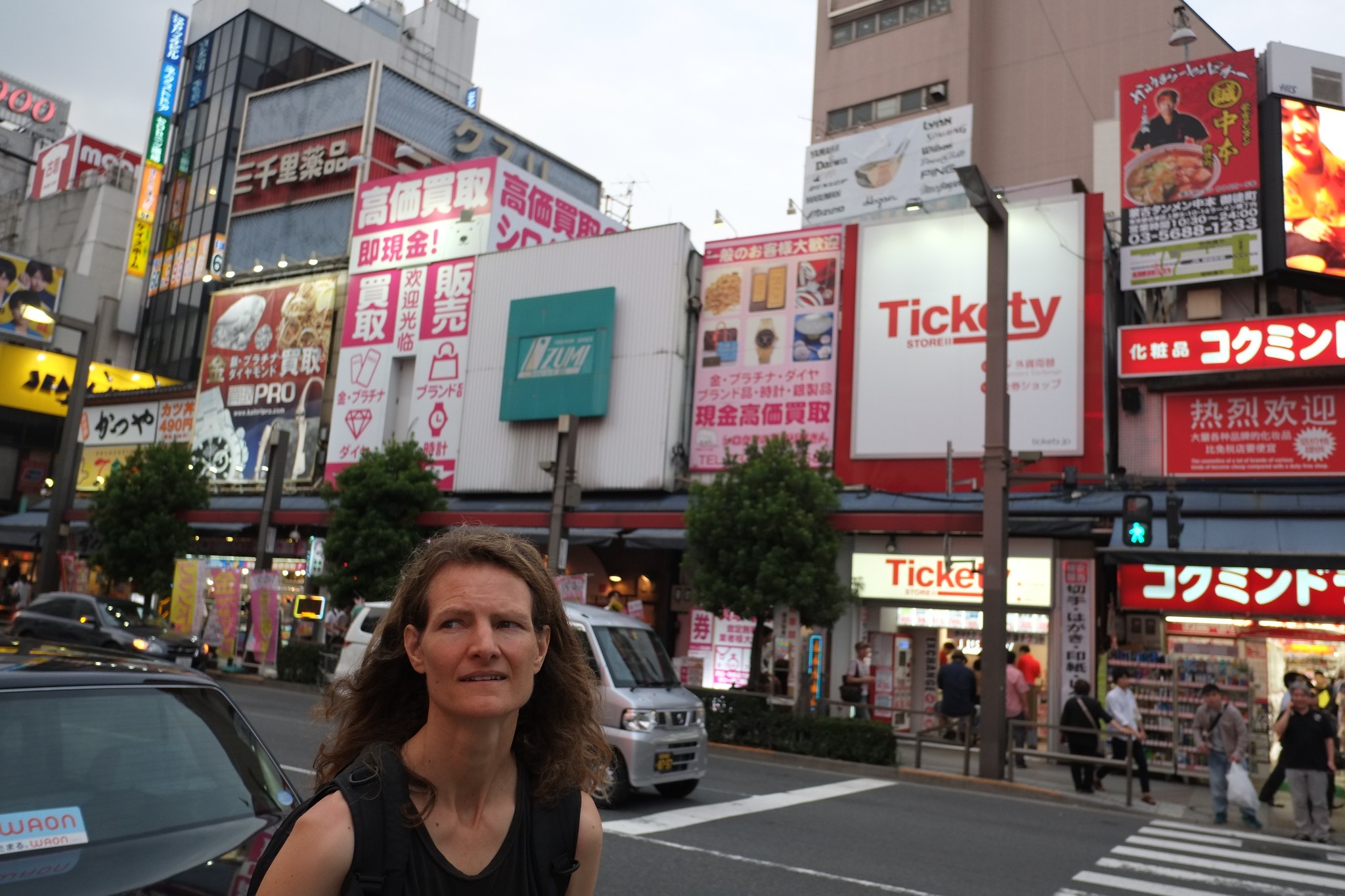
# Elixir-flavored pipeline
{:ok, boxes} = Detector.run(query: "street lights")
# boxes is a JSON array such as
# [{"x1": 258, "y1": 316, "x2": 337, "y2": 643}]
[
  {"x1": 955, "y1": 163, "x2": 1008, "y2": 781},
  {"x1": 19, "y1": 301, "x2": 101, "y2": 603}
]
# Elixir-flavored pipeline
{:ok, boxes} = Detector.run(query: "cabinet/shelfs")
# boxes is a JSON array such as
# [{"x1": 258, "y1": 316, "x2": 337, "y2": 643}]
[{"x1": 1105, "y1": 651, "x2": 1274, "y2": 786}]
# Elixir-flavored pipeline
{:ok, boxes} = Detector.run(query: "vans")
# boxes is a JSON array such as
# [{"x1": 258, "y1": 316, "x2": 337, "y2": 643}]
[{"x1": 337, "y1": 600, "x2": 707, "y2": 810}]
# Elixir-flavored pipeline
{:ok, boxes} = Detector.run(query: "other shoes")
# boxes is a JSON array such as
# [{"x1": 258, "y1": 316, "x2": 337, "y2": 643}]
[
  {"x1": 1319, "y1": 837, "x2": 1337, "y2": 846},
  {"x1": 1294, "y1": 834, "x2": 1311, "y2": 841},
  {"x1": 1143, "y1": 796, "x2": 1157, "y2": 805},
  {"x1": 1242, "y1": 814, "x2": 1262, "y2": 830},
  {"x1": 1093, "y1": 776, "x2": 1104, "y2": 792},
  {"x1": 1260, "y1": 794, "x2": 1285, "y2": 808},
  {"x1": 1017, "y1": 760, "x2": 1028, "y2": 769},
  {"x1": 1213, "y1": 811, "x2": 1227, "y2": 825}
]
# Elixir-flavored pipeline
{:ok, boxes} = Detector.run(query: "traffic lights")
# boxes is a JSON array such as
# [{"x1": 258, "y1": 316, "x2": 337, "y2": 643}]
[
  {"x1": 1121, "y1": 496, "x2": 1153, "y2": 548},
  {"x1": 1166, "y1": 486, "x2": 1185, "y2": 549}
]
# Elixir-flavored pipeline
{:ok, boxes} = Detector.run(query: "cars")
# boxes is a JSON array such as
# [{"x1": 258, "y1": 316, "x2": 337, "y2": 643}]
[{"x1": 0, "y1": 633, "x2": 305, "y2": 896}]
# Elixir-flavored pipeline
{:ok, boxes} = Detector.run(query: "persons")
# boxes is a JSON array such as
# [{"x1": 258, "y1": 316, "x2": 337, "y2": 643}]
[
  {"x1": 0, "y1": 256, "x2": 55, "y2": 339},
  {"x1": 246, "y1": 524, "x2": 605, "y2": 896},
  {"x1": 1281, "y1": 99, "x2": 1345, "y2": 278},
  {"x1": 833, "y1": 641, "x2": 1345, "y2": 844},
  {"x1": 323, "y1": 597, "x2": 351, "y2": 648},
  {"x1": 12, "y1": 573, "x2": 33, "y2": 612},
  {"x1": 1129, "y1": 89, "x2": 1209, "y2": 156}
]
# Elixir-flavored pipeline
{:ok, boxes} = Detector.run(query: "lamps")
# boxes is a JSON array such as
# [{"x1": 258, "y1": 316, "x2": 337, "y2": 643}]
[
  {"x1": 203, "y1": 251, "x2": 335, "y2": 283},
  {"x1": 992, "y1": 186, "x2": 1008, "y2": 204},
  {"x1": 104, "y1": 370, "x2": 181, "y2": 388},
  {"x1": 45, "y1": 121, "x2": 75, "y2": 139},
  {"x1": 886, "y1": 534, "x2": 897, "y2": 553},
  {"x1": 715, "y1": 210, "x2": 738, "y2": 239},
  {"x1": 346, "y1": 143, "x2": 456, "y2": 174},
  {"x1": 906, "y1": 198, "x2": 929, "y2": 213},
  {"x1": 290, "y1": 524, "x2": 301, "y2": 539},
  {"x1": 787, "y1": 199, "x2": 812, "y2": 227},
  {"x1": 1168, "y1": 6, "x2": 1197, "y2": 48}
]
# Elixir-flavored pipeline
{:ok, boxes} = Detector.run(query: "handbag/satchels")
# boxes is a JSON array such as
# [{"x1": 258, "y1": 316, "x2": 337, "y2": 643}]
[
  {"x1": 838, "y1": 661, "x2": 861, "y2": 702},
  {"x1": 1226, "y1": 760, "x2": 1260, "y2": 811}
]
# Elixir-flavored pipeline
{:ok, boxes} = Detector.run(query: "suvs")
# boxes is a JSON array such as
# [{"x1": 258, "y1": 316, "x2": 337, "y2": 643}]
[{"x1": 11, "y1": 591, "x2": 209, "y2": 674}]
